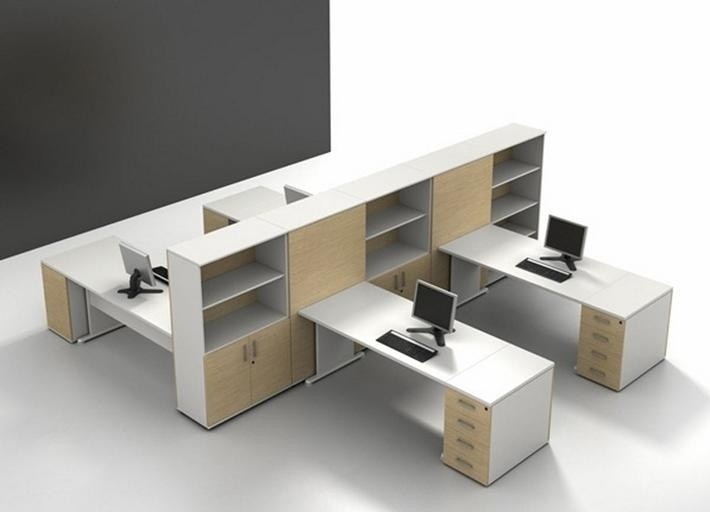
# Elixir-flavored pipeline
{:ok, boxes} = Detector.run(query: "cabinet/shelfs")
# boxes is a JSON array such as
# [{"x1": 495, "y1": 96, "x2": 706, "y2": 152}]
[{"x1": 166, "y1": 123, "x2": 546, "y2": 430}]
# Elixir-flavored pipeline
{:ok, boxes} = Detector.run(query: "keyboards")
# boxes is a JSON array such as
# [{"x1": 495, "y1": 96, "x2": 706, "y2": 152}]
[
  {"x1": 515, "y1": 257, "x2": 571, "y2": 284},
  {"x1": 376, "y1": 330, "x2": 438, "y2": 362},
  {"x1": 152, "y1": 265, "x2": 169, "y2": 283}
]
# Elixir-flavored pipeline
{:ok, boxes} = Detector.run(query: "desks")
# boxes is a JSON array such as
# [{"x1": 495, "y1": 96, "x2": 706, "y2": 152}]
[
  {"x1": 41, "y1": 235, "x2": 174, "y2": 353},
  {"x1": 201, "y1": 187, "x2": 286, "y2": 234},
  {"x1": 298, "y1": 281, "x2": 555, "y2": 487},
  {"x1": 438, "y1": 224, "x2": 673, "y2": 393}
]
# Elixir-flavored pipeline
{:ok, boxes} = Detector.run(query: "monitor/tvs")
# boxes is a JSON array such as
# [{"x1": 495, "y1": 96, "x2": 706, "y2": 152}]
[
  {"x1": 540, "y1": 214, "x2": 587, "y2": 271},
  {"x1": 406, "y1": 278, "x2": 458, "y2": 347},
  {"x1": 284, "y1": 184, "x2": 311, "y2": 204},
  {"x1": 117, "y1": 241, "x2": 163, "y2": 298}
]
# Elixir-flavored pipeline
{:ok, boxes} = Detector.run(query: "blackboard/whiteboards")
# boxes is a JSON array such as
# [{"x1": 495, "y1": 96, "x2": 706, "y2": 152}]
[{"x1": 0, "y1": 0, "x2": 332, "y2": 261}]
